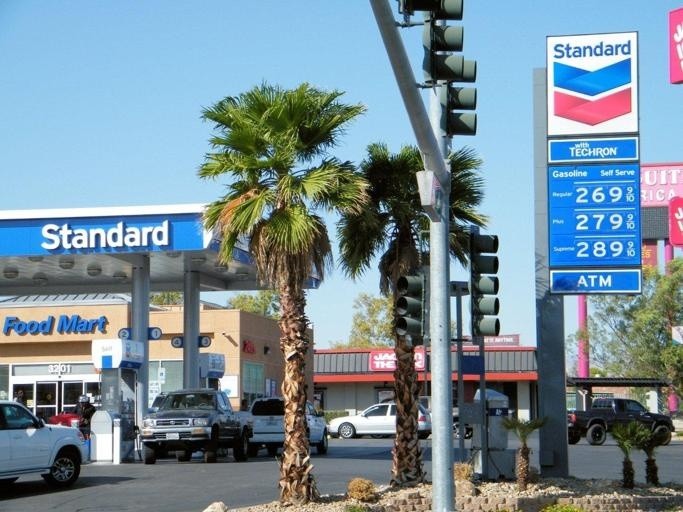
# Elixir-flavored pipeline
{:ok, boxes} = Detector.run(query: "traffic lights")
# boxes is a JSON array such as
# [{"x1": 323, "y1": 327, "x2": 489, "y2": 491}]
[
  {"x1": 393, "y1": 272, "x2": 428, "y2": 337},
  {"x1": 395, "y1": 0, "x2": 477, "y2": 136},
  {"x1": 470, "y1": 234, "x2": 500, "y2": 336}
]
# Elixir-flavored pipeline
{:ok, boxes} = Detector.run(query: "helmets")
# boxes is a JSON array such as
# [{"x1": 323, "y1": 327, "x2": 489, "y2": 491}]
[{"x1": 78, "y1": 395, "x2": 89, "y2": 403}]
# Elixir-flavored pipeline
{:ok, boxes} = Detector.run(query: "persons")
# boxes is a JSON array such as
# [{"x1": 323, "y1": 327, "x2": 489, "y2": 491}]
[
  {"x1": 45, "y1": 392, "x2": 60, "y2": 415},
  {"x1": 72, "y1": 396, "x2": 96, "y2": 419},
  {"x1": 14, "y1": 390, "x2": 26, "y2": 417}
]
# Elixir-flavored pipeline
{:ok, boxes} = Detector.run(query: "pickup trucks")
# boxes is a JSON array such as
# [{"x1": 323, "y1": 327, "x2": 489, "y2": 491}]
[
  {"x1": 568, "y1": 398, "x2": 675, "y2": 445},
  {"x1": 139, "y1": 388, "x2": 254, "y2": 464}
]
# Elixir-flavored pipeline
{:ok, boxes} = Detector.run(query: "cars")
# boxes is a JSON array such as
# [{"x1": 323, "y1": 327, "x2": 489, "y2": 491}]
[
  {"x1": 151, "y1": 391, "x2": 167, "y2": 413},
  {"x1": 247, "y1": 397, "x2": 473, "y2": 458},
  {"x1": 0, "y1": 400, "x2": 88, "y2": 488}
]
